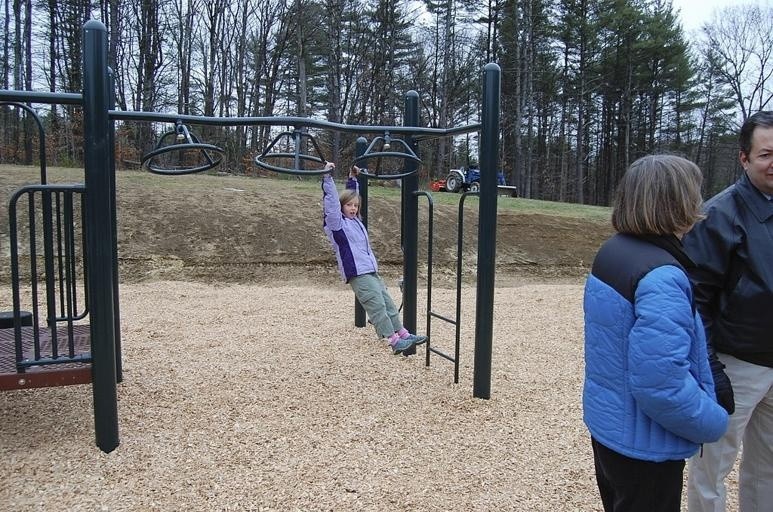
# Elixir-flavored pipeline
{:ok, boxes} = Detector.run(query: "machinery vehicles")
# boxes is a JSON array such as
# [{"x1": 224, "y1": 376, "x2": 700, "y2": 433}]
[{"x1": 428, "y1": 150, "x2": 518, "y2": 199}]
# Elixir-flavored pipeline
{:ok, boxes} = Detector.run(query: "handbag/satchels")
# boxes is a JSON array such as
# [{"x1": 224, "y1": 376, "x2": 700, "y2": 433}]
[{"x1": 707, "y1": 354, "x2": 735, "y2": 415}]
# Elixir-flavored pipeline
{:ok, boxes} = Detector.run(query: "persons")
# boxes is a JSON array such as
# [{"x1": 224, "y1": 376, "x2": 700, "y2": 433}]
[
  {"x1": 679, "y1": 108, "x2": 772, "y2": 512},
  {"x1": 582, "y1": 154, "x2": 731, "y2": 512},
  {"x1": 322, "y1": 163, "x2": 427, "y2": 355}
]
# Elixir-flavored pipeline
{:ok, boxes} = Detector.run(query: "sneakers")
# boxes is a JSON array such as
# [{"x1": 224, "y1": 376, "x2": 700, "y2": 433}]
[
  {"x1": 402, "y1": 333, "x2": 428, "y2": 346},
  {"x1": 388, "y1": 336, "x2": 418, "y2": 355}
]
